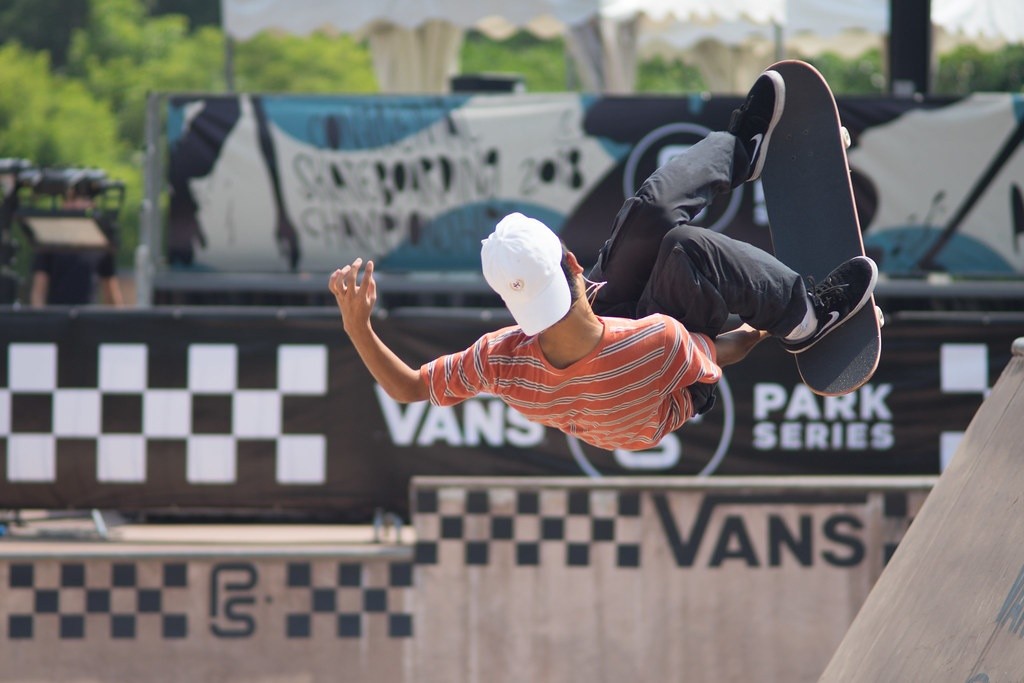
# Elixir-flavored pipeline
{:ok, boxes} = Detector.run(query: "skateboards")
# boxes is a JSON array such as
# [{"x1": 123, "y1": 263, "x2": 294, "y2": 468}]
[{"x1": 749, "y1": 58, "x2": 885, "y2": 396}]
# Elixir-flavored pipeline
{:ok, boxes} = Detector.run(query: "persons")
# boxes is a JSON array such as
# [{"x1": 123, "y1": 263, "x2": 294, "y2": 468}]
[
  {"x1": 0, "y1": 156, "x2": 127, "y2": 309},
  {"x1": 329, "y1": 71, "x2": 878, "y2": 452}
]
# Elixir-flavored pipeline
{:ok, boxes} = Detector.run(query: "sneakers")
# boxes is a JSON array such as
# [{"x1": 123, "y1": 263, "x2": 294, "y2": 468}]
[
  {"x1": 729, "y1": 70, "x2": 785, "y2": 181},
  {"x1": 780, "y1": 256, "x2": 879, "y2": 354}
]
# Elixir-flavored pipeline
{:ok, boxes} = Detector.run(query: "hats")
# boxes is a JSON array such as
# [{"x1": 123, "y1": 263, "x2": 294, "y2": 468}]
[{"x1": 480, "y1": 212, "x2": 571, "y2": 336}]
